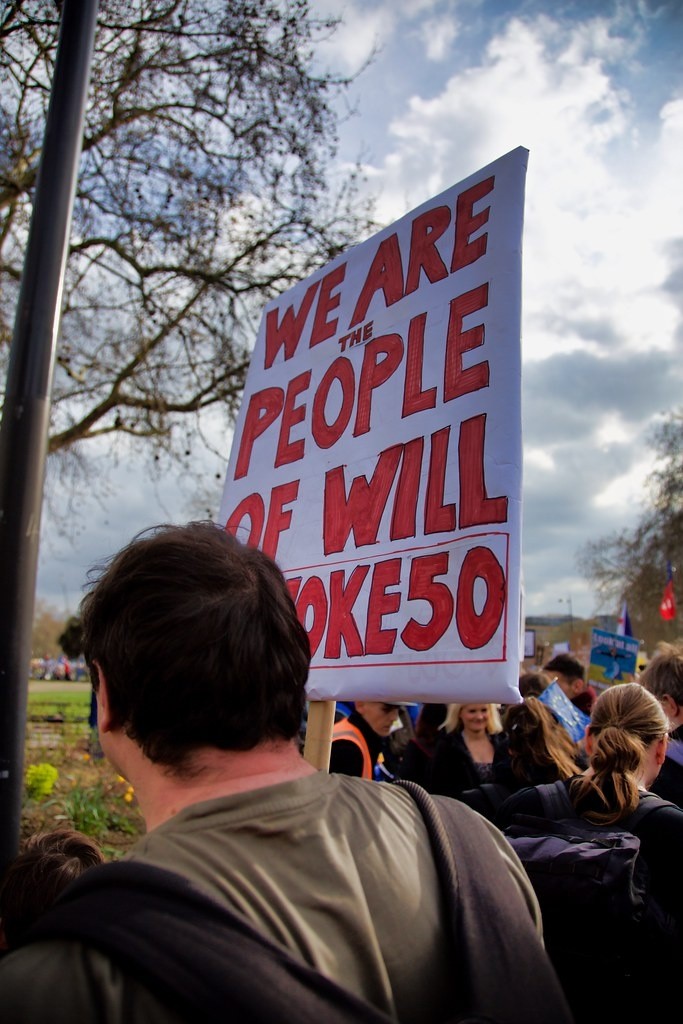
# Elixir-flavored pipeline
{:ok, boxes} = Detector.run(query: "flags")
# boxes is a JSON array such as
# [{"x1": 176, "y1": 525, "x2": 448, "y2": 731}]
[{"x1": 658, "y1": 561, "x2": 678, "y2": 622}]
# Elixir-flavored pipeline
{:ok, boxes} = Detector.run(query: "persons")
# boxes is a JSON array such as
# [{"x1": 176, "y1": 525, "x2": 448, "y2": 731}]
[
  {"x1": 0, "y1": 523, "x2": 577, "y2": 1024},
  {"x1": 0, "y1": 828, "x2": 104, "y2": 955},
  {"x1": 501, "y1": 681, "x2": 683, "y2": 1024},
  {"x1": 297, "y1": 652, "x2": 683, "y2": 819}
]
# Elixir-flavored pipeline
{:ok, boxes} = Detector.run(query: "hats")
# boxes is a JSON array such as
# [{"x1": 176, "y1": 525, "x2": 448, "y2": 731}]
[
  {"x1": 384, "y1": 702, "x2": 417, "y2": 707},
  {"x1": 543, "y1": 653, "x2": 585, "y2": 680}
]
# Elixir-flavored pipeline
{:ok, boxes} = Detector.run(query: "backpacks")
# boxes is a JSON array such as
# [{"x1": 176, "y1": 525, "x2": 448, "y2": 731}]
[{"x1": 501, "y1": 780, "x2": 683, "y2": 1024}]
